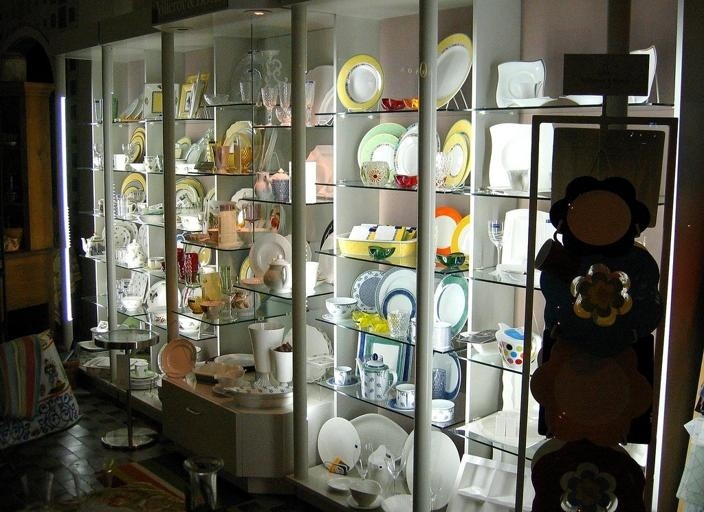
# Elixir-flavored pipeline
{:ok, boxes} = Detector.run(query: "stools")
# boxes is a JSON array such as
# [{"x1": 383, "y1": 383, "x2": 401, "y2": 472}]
[{"x1": 93, "y1": 328, "x2": 161, "y2": 452}]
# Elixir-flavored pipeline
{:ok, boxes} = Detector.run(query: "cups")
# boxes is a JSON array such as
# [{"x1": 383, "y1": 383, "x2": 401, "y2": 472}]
[
  {"x1": 195, "y1": 345, "x2": 202, "y2": 359},
  {"x1": 278, "y1": 81, "x2": 291, "y2": 124},
  {"x1": 134, "y1": 191, "x2": 146, "y2": 210},
  {"x1": 144, "y1": 156, "x2": 157, "y2": 172},
  {"x1": 360, "y1": 161, "x2": 390, "y2": 186},
  {"x1": 435, "y1": 151, "x2": 454, "y2": 188},
  {"x1": 262, "y1": 87, "x2": 278, "y2": 126},
  {"x1": 125, "y1": 202, "x2": 137, "y2": 215},
  {"x1": 213, "y1": 146, "x2": 230, "y2": 173},
  {"x1": 395, "y1": 384, "x2": 416, "y2": 409},
  {"x1": 94, "y1": 98, "x2": 102, "y2": 122},
  {"x1": 148, "y1": 257, "x2": 164, "y2": 268},
  {"x1": 508, "y1": 170, "x2": 528, "y2": 190},
  {"x1": 122, "y1": 143, "x2": 132, "y2": 168},
  {"x1": 94, "y1": 143, "x2": 104, "y2": 167},
  {"x1": 271, "y1": 347, "x2": 294, "y2": 383},
  {"x1": 387, "y1": 310, "x2": 410, "y2": 339},
  {"x1": 488, "y1": 221, "x2": 504, "y2": 273},
  {"x1": 335, "y1": 366, "x2": 352, "y2": 385},
  {"x1": 305, "y1": 81, "x2": 316, "y2": 125},
  {"x1": 114, "y1": 154, "x2": 130, "y2": 170},
  {"x1": 134, "y1": 360, "x2": 148, "y2": 376},
  {"x1": 116, "y1": 288, "x2": 127, "y2": 309},
  {"x1": 117, "y1": 196, "x2": 127, "y2": 217},
  {"x1": 239, "y1": 81, "x2": 252, "y2": 101}
]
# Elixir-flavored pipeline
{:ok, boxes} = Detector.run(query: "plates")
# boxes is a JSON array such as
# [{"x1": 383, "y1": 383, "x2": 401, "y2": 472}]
[
  {"x1": 318, "y1": 232, "x2": 334, "y2": 285},
  {"x1": 202, "y1": 189, "x2": 215, "y2": 215},
  {"x1": 231, "y1": 188, "x2": 258, "y2": 227},
  {"x1": 503, "y1": 358, "x2": 542, "y2": 421},
  {"x1": 337, "y1": 55, "x2": 384, "y2": 111},
  {"x1": 306, "y1": 145, "x2": 334, "y2": 200},
  {"x1": 350, "y1": 414, "x2": 409, "y2": 478},
  {"x1": 212, "y1": 383, "x2": 232, "y2": 399},
  {"x1": 130, "y1": 162, "x2": 146, "y2": 172},
  {"x1": 127, "y1": 136, "x2": 144, "y2": 161},
  {"x1": 194, "y1": 364, "x2": 245, "y2": 381},
  {"x1": 187, "y1": 71, "x2": 211, "y2": 119},
  {"x1": 173, "y1": 176, "x2": 204, "y2": 215},
  {"x1": 177, "y1": 234, "x2": 185, "y2": 249},
  {"x1": 355, "y1": 333, "x2": 412, "y2": 386},
  {"x1": 376, "y1": 267, "x2": 418, "y2": 321},
  {"x1": 285, "y1": 232, "x2": 312, "y2": 261},
  {"x1": 434, "y1": 206, "x2": 462, "y2": 255},
  {"x1": 240, "y1": 256, "x2": 255, "y2": 279},
  {"x1": 347, "y1": 495, "x2": 383, "y2": 510},
  {"x1": 565, "y1": 45, "x2": 656, "y2": 105},
  {"x1": 158, "y1": 343, "x2": 167, "y2": 374},
  {"x1": 401, "y1": 431, "x2": 461, "y2": 511},
  {"x1": 434, "y1": 351, "x2": 461, "y2": 402},
  {"x1": 130, "y1": 144, "x2": 141, "y2": 161},
  {"x1": 433, "y1": 274, "x2": 469, "y2": 337},
  {"x1": 178, "y1": 80, "x2": 208, "y2": 118},
  {"x1": 146, "y1": 280, "x2": 182, "y2": 316},
  {"x1": 198, "y1": 247, "x2": 212, "y2": 266},
  {"x1": 497, "y1": 60, "x2": 544, "y2": 110},
  {"x1": 457, "y1": 411, "x2": 547, "y2": 452},
  {"x1": 407, "y1": 123, "x2": 441, "y2": 175},
  {"x1": 144, "y1": 82, "x2": 179, "y2": 119},
  {"x1": 489, "y1": 122, "x2": 553, "y2": 191},
  {"x1": 382, "y1": 289, "x2": 417, "y2": 322},
  {"x1": 382, "y1": 494, "x2": 414, "y2": 512},
  {"x1": 488, "y1": 187, "x2": 532, "y2": 197},
  {"x1": 102, "y1": 220, "x2": 138, "y2": 251},
  {"x1": 362, "y1": 134, "x2": 399, "y2": 185},
  {"x1": 357, "y1": 123, "x2": 407, "y2": 183},
  {"x1": 177, "y1": 137, "x2": 192, "y2": 158},
  {"x1": 133, "y1": 127, "x2": 145, "y2": 139},
  {"x1": 144, "y1": 268, "x2": 163, "y2": 273},
  {"x1": 121, "y1": 173, "x2": 146, "y2": 202},
  {"x1": 271, "y1": 204, "x2": 286, "y2": 233},
  {"x1": 321, "y1": 314, "x2": 354, "y2": 323},
  {"x1": 129, "y1": 89, "x2": 144, "y2": 119},
  {"x1": 130, "y1": 132, "x2": 146, "y2": 162},
  {"x1": 110, "y1": 226, "x2": 131, "y2": 248},
  {"x1": 162, "y1": 338, "x2": 197, "y2": 378},
  {"x1": 243, "y1": 280, "x2": 262, "y2": 287},
  {"x1": 395, "y1": 134, "x2": 418, "y2": 178},
  {"x1": 451, "y1": 214, "x2": 470, "y2": 269},
  {"x1": 223, "y1": 121, "x2": 262, "y2": 170},
  {"x1": 437, "y1": 33, "x2": 473, "y2": 110},
  {"x1": 283, "y1": 324, "x2": 333, "y2": 383},
  {"x1": 249, "y1": 233, "x2": 292, "y2": 277},
  {"x1": 137, "y1": 214, "x2": 163, "y2": 224},
  {"x1": 388, "y1": 398, "x2": 415, "y2": 412},
  {"x1": 120, "y1": 98, "x2": 139, "y2": 118},
  {"x1": 306, "y1": 65, "x2": 333, "y2": 125},
  {"x1": 443, "y1": 119, "x2": 472, "y2": 188},
  {"x1": 214, "y1": 354, "x2": 257, "y2": 368},
  {"x1": 351, "y1": 270, "x2": 387, "y2": 315},
  {"x1": 124, "y1": 187, "x2": 137, "y2": 198},
  {"x1": 328, "y1": 476, "x2": 354, "y2": 494},
  {"x1": 326, "y1": 377, "x2": 358, "y2": 388},
  {"x1": 443, "y1": 132, "x2": 467, "y2": 187},
  {"x1": 317, "y1": 417, "x2": 361, "y2": 473}
]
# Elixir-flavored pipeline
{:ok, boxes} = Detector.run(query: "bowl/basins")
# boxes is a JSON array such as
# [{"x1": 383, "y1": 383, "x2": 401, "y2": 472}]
[
  {"x1": 174, "y1": 159, "x2": 187, "y2": 168},
  {"x1": 403, "y1": 98, "x2": 419, "y2": 110},
  {"x1": 235, "y1": 292, "x2": 260, "y2": 316},
  {"x1": 203, "y1": 93, "x2": 230, "y2": 105},
  {"x1": 200, "y1": 301, "x2": 224, "y2": 320},
  {"x1": 437, "y1": 253, "x2": 465, "y2": 269},
  {"x1": 305, "y1": 261, "x2": 320, "y2": 292},
  {"x1": 459, "y1": 331, "x2": 498, "y2": 353},
  {"x1": 349, "y1": 479, "x2": 383, "y2": 506},
  {"x1": 326, "y1": 297, "x2": 358, "y2": 317},
  {"x1": 188, "y1": 298, "x2": 205, "y2": 314},
  {"x1": 430, "y1": 399, "x2": 455, "y2": 422},
  {"x1": 338, "y1": 234, "x2": 418, "y2": 258},
  {"x1": 368, "y1": 246, "x2": 395, "y2": 261},
  {"x1": 122, "y1": 296, "x2": 142, "y2": 313},
  {"x1": 225, "y1": 386, "x2": 296, "y2": 410},
  {"x1": 393, "y1": 173, "x2": 418, "y2": 187},
  {"x1": 276, "y1": 109, "x2": 293, "y2": 125},
  {"x1": 381, "y1": 98, "x2": 405, "y2": 111},
  {"x1": 507, "y1": 97, "x2": 549, "y2": 108},
  {"x1": 207, "y1": 229, "x2": 219, "y2": 241}
]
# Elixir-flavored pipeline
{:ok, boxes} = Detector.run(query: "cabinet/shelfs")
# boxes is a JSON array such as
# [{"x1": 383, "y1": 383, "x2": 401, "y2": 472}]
[{"x1": 46, "y1": 0, "x2": 703, "y2": 512}]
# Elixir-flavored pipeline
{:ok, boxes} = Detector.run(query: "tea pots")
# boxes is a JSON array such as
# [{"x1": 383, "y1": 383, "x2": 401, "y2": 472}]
[
  {"x1": 368, "y1": 444, "x2": 395, "y2": 496},
  {"x1": 81, "y1": 233, "x2": 105, "y2": 258},
  {"x1": 496, "y1": 322, "x2": 541, "y2": 367},
  {"x1": 357, "y1": 354, "x2": 398, "y2": 403},
  {"x1": 263, "y1": 253, "x2": 292, "y2": 295},
  {"x1": 266, "y1": 168, "x2": 290, "y2": 202},
  {"x1": 254, "y1": 172, "x2": 272, "y2": 200},
  {"x1": 126, "y1": 239, "x2": 146, "y2": 269}
]
